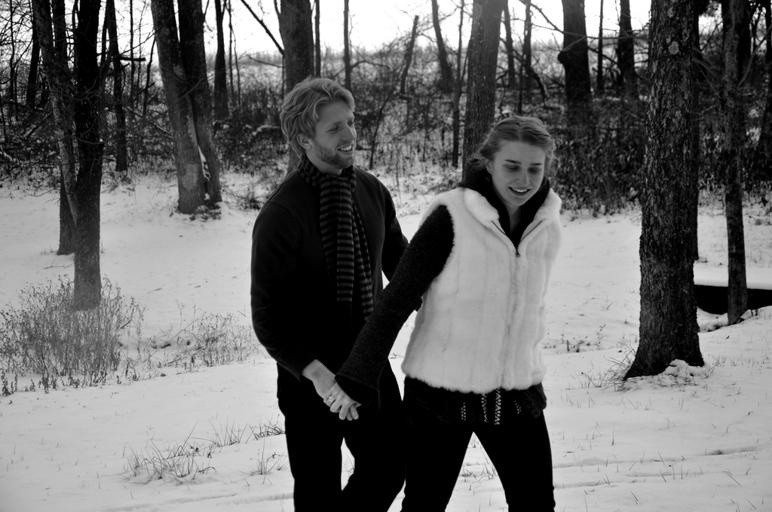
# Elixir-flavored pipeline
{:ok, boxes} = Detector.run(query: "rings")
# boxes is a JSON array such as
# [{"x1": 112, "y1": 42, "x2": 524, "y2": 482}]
[{"x1": 328, "y1": 396, "x2": 334, "y2": 402}]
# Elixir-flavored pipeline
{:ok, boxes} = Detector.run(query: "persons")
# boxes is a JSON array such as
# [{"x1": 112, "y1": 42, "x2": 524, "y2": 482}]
[
  {"x1": 322, "y1": 115, "x2": 563, "y2": 512},
  {"x1": 248, "y1": 77, "x2": 424, "y2": 512}
]
